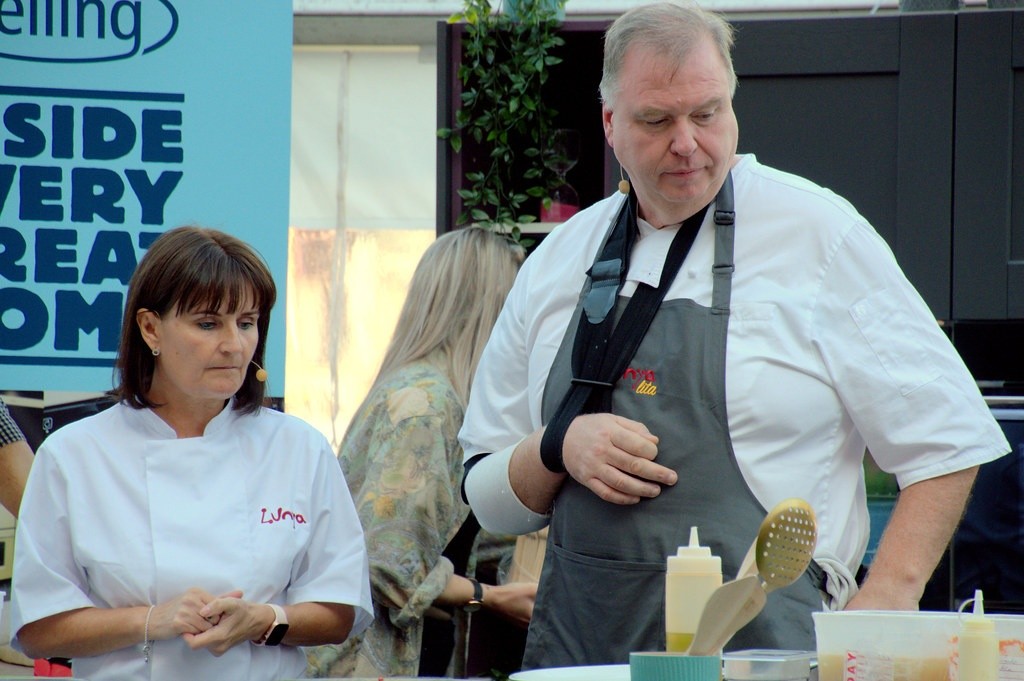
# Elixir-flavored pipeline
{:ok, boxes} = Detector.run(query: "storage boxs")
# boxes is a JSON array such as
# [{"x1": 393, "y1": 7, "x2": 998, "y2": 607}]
[{"x1": 811, "y1": 610, "x2": 1024, "y2": 681}]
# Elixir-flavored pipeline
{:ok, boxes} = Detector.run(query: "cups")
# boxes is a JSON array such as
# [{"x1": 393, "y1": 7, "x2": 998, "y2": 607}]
[{"x1": 629, "y1": 651, "x2": 720, "y2": 681}]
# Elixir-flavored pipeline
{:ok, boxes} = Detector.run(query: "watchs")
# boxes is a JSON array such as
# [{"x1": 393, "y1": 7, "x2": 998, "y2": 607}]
[
  {"x1": 463, "y1": 579, "x2": 483, "y2": 613},
  {"x1": 251, "y1": 603, "x2": 289, "y2": 646}
]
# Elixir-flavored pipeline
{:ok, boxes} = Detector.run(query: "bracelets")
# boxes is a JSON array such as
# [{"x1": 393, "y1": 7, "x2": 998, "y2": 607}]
[{"x1": 143, "y1": 605, "x2": 156, "y2": 663}]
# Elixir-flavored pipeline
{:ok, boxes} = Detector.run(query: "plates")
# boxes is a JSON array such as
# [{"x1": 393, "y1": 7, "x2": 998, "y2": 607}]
[{"x1": 509, "y1": 664, "x2": 631, "y2": 681}]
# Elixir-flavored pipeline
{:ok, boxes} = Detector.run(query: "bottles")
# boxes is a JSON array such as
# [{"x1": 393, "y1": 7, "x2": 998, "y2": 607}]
[
  {"x1": 666, "y1": 526, "x2": 724, "y2": 653},
  {"x1": 956, "y1": 590, "x2": 1000, "y2": 681}
]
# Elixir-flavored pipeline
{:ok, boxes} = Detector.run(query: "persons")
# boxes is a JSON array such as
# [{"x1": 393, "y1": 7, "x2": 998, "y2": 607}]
[
  {"x1": 9, "y1": 226, "x2": 374, "y2": 681},
  {"x1": 0, "y1": 399, "x2": 36, "y2": 519},
  {"x1": 457, "y1": 3, "x2": 1011, "y2": 671},
  {"x1": 297, "y1": 227, "x2": 543, "y2": 681}
]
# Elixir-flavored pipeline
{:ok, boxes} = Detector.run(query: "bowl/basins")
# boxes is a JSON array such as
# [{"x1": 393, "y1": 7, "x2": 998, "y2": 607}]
[{"x1": 721, "y1": 649, "x2": 818, "y2": 681}]
